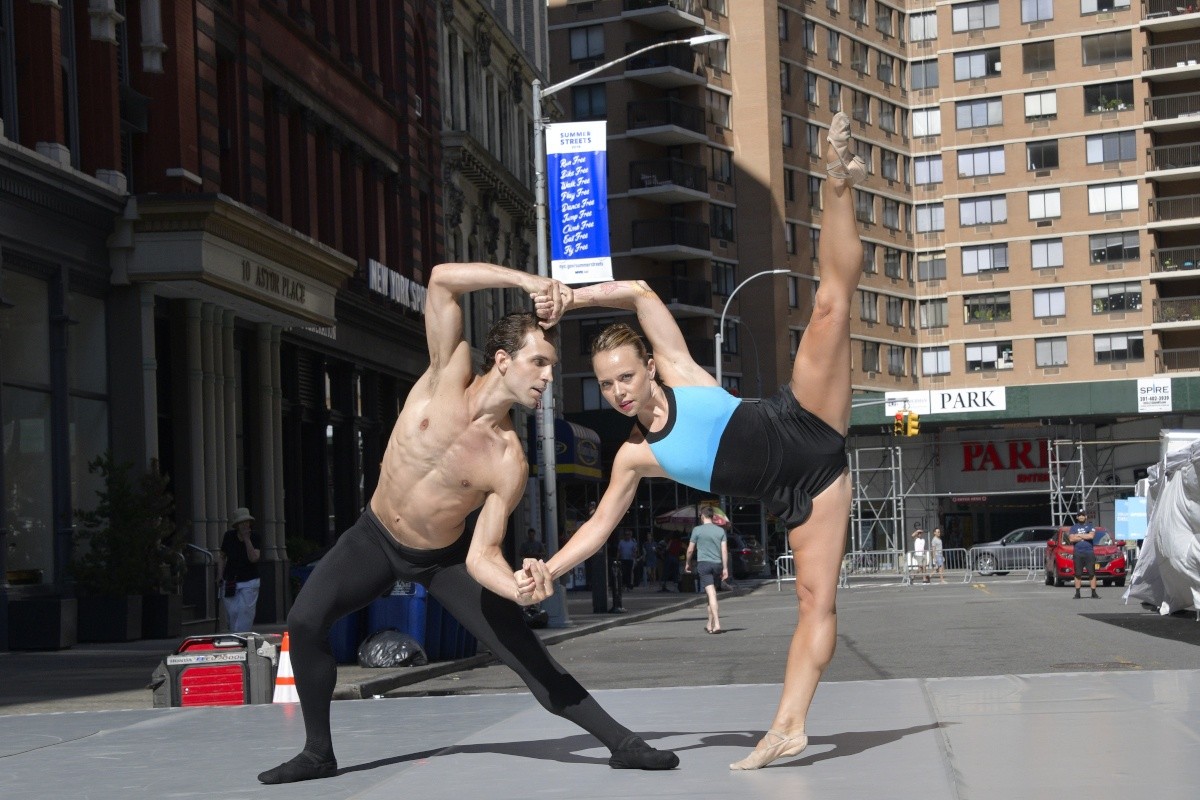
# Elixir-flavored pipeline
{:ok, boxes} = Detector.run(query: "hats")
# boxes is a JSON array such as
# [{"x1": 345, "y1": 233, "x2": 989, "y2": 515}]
[
  {"x1": 1078, "y1": 509, "x2": 1085, "y2": 515},
  {"x1": 230, "y1": 508, "x2": 255, "y2": 527}
]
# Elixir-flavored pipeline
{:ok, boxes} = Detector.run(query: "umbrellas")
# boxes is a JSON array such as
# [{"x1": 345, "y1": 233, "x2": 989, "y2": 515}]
[{"x1": 656, "y1": 505, "x2": 730, "y2": 535}]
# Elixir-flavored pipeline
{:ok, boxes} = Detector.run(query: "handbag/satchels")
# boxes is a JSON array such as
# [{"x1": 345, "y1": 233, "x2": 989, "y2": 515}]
[{"x1": 523, "y1": 606, "x2": 549, "y2": 628}]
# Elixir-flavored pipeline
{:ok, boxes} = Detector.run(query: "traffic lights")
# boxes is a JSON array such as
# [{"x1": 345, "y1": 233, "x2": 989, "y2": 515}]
[
  {"x1": 906, "y1": 411, "x2": 921, "y2": 437},
  {"x1": 894, "y1": 412, "x2": 904, "y2": 436}
]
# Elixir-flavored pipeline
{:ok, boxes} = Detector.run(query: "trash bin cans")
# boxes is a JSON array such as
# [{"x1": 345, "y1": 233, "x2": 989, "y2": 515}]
[
  {"x1": 147, "y1": 632, "x2": 282, "y2": 709},
  {"x1": 286, "y1": 567, "x2": 476, "y2": 660}
]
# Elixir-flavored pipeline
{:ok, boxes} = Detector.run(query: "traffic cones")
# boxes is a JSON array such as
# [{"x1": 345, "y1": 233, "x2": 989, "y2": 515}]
[{"x1": 272, "y1": 632, "x2": 304, "y2": 706}]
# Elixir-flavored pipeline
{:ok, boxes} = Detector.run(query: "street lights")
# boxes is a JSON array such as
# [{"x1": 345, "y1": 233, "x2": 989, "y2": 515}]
[
  {"x1": 715, "y1": 268, "x2": 792, "y2": 387},
  {"x1": 532, "y1": 32, "x2": 733, "y2": 629}
]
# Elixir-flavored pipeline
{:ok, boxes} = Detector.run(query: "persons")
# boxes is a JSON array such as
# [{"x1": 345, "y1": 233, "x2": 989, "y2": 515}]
[
  {"x1": 557, "y1": 506, "x2": 945, "y2": 635},
  {"x1": 255, "y1": 261, "x2": 678, "y2": 784},
  {"x1": 520, "y1": 528, "x2": 545, "y2": 563},
  {"x1": 518, "y1": 111, "x2": 864, "y2": 769},
  {"x1": 217, "y1": 509, "x2": 263, "y2": 634},
  {"x1": 1069, "y1": 509, "x2": 1099, "y2": 599}
]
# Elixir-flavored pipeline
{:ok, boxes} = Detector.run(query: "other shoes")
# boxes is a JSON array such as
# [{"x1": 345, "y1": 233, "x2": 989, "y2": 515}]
[
  {"x1": 258, "y1": 750, "x2": 338, "y2": 785},
  {"x1": 1073, "y1": 593, "x2": 1080, "y2": 599},
  {"x1": 609, "y1": 735, "x2": 680, "y2": 771},
  {"x1": 1092, "y1": 593, "x2": 1099, "y2": 598}
]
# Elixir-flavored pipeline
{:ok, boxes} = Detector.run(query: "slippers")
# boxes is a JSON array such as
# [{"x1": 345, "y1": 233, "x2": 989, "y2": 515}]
[{"x1": 704, "y1": 627, "x2": 723, "y2": 634}]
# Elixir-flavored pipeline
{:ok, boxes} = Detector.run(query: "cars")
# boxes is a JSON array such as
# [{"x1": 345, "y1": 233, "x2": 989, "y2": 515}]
[
  {"x1": 726, "y1": 533, "x2": 767, "y2": 580},
  {"x1": 968, "y1": 525, "x2": 1069, "y2": 576},
  {"x1": 1044, "y1": 527, "x2": 1128, "y2": 587}
]
